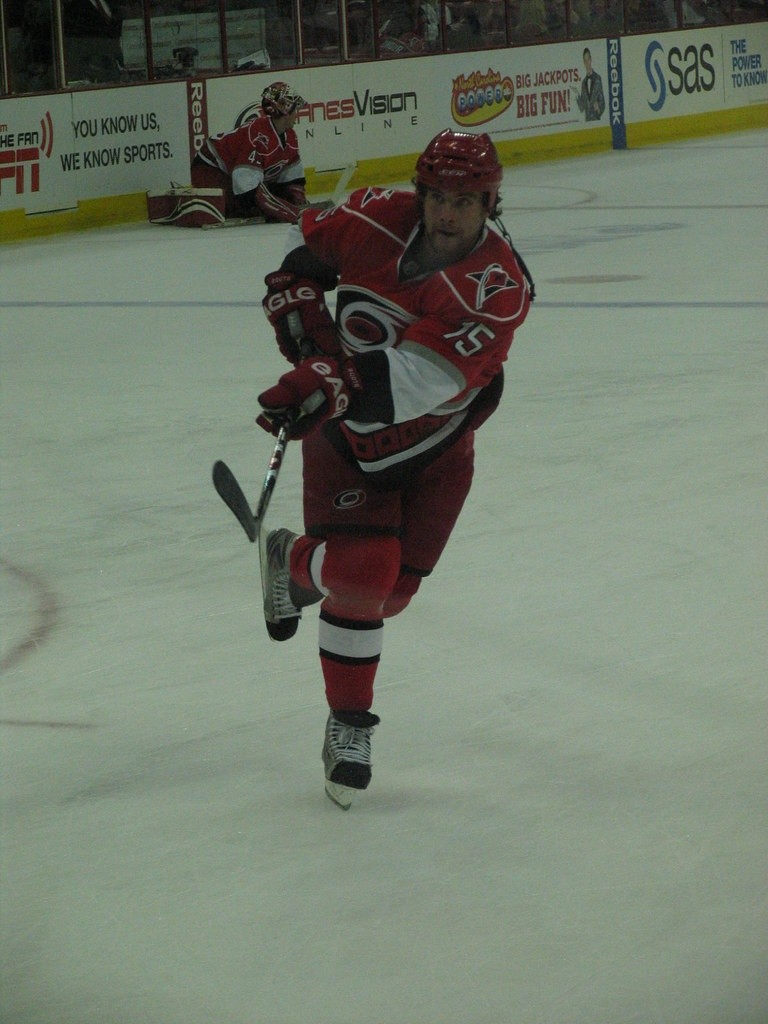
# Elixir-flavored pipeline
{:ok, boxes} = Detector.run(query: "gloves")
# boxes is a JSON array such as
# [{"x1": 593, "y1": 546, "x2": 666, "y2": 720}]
[
  {"x1": 256, "y1": 354, "x2": 371, "y2": 443},
  {"x1": 261, "y1": 281, "x2": 342, "y2": 364}
]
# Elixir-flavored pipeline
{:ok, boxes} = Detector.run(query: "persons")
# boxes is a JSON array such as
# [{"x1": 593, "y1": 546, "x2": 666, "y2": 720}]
[
  {"x1": 255, "y1": 129, "x2": 534, "y2": 812},
  {"x1": 570, "y1": 48, "x2": 605, "y2": 121},
  {"x1": 146, "y1": 82, "x2": 310, "y2": 228},
  {"x1": 0, "y1": 0, "x2": 768, "y2": 87}
]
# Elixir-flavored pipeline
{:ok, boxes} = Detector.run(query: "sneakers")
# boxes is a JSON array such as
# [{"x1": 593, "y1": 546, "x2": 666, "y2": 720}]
[
  {"x1": 258, "y1": 525, "x2": 302, "y2": 646},
  {"x1": 322, "y1": 710, "x2": 381, "y2": 810}
]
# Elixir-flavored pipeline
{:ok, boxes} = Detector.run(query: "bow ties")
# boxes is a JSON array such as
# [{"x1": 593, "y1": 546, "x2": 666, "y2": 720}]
[{"x1": 585, "y1": 75, "x2": 592, "y2": 79}]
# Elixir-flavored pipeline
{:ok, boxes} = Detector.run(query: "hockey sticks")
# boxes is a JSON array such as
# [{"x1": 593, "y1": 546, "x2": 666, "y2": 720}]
[
  {"x1": 212, "y1": 341, "x2": 313, "y2": 543},
  {"x1": 202, "y1": 159, "x2": 357, "y2": 231}
]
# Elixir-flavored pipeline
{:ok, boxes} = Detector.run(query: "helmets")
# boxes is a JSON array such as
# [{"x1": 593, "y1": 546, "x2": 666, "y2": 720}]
[
  {"x1": 415, "y1": 127, "x2": 502, "y2": 194},
  {"x1": 261, "y1": 82, "x2": 308, "y2": 117}
]
